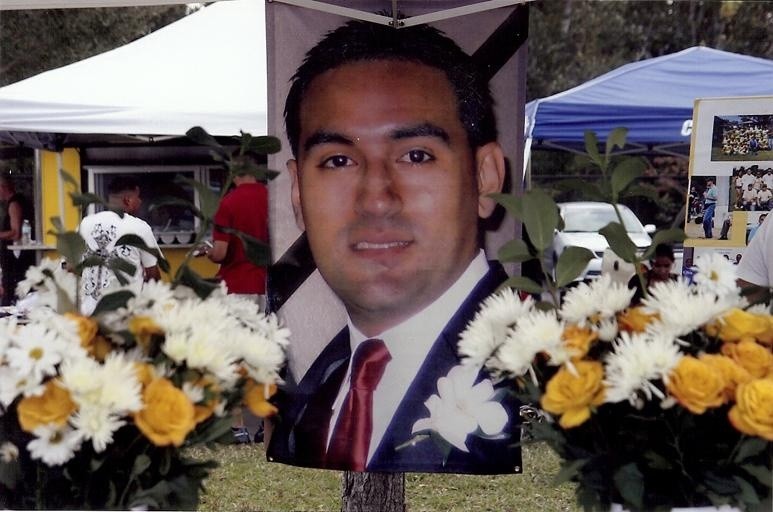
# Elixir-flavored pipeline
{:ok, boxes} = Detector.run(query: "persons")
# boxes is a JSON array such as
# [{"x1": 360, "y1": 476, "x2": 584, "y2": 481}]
[
  {"x1": 732, "y1": 207, "x2": 773, "y2": 315},
  {"x1": 0, "y1": 169, "x2": 33, "y2": 317},
  {"x1": 689, "y1": 121, "x2": 773, "y2": 247},
  {"x1": 628, "y1": 242, "x2": 679, "y2": 309},
  {"x1": 261, "y1": 10, "x2": 524, "y2": 475},
  {"x1": 78, "y1": 175, "x2": 161, "y2": 308},
  {"x1": 206, "y1": 146, "x2": 269, "y2": 296}
]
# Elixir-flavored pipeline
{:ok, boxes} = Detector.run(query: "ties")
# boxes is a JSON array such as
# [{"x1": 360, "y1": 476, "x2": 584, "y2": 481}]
[{"x1": 326, "y1": 337, "x2": 391, "y2": 474}]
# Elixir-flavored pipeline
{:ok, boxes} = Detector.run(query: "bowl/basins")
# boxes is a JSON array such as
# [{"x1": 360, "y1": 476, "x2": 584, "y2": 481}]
[{"x1": 152, "y1": 231, "x2": 194, "y2": 245}]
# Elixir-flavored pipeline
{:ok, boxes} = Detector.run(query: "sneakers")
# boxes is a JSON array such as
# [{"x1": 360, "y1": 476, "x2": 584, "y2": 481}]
[{"x1": 213, "y1": 420, "x2": 264, "y2": 444}]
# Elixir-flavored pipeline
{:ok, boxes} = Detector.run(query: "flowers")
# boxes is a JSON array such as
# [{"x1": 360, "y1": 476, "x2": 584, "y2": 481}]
[
  {"x1": 0, "y1": 125, "x2": 293, "y2": 505},
  {"x1": 404, "y1": 117, "x2": 771, "y2": 507}
]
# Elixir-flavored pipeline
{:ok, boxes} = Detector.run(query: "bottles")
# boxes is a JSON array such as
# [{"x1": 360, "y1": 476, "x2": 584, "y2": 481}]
[{"x1": 21, "y1": 219, "x2": 31, "y2": 246}]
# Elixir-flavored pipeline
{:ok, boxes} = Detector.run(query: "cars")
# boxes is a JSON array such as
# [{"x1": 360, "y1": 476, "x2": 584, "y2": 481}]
[{"x1": 547, "y1": 200, "x2": 655, "y2": 302}]
